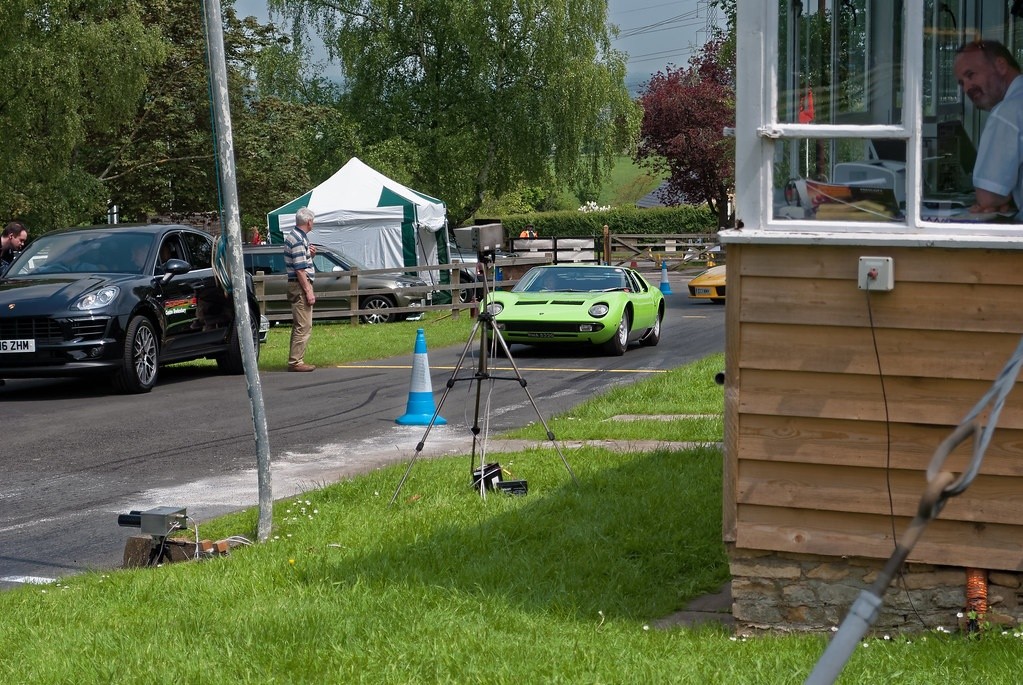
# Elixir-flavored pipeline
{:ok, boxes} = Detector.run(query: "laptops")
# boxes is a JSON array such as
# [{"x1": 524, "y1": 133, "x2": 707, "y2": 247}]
[{"x1": 868, "y1": 140, "x2": 975, "y2": 208}]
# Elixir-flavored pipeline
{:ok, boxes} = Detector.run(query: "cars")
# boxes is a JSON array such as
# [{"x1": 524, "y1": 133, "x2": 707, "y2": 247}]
[
  {"x1": 898, "y1": 190, "x2": 978, "y2": 219},
  {"x1": 0, "y1": 222, "x2": 262, "y2": 392}
]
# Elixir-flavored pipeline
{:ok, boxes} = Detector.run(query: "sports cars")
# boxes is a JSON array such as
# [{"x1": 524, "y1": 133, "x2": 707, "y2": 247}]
[
  {"x1": 686, "y1": 264, "x2": 728, "y2": 305},
  {"x1": 478, "y1": 265, "x2": 665, "y2": 357}
]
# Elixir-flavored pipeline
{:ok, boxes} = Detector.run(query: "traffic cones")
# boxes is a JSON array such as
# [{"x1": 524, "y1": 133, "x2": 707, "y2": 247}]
[
  {"x1": 394, "y1": 327, "x2": 449, "y2": 425},
  {"x1": 659, "y1": 258, "x2": 673, "y2": 295}
]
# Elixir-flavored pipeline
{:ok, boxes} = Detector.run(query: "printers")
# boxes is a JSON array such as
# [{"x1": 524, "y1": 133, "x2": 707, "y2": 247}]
[{"x1": 833, "y1": 159, "x2": 906, "y2": 209}]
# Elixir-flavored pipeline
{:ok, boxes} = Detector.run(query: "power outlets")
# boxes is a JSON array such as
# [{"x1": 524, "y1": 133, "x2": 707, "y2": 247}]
[{"x1": 857, "y1": 257, "x2": 894, "y2": 290}]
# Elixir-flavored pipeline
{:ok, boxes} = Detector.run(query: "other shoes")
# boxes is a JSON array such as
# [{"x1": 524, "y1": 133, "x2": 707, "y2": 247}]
[{"x1": 288, "y1": 362, "x2": 316, "y2": 371}]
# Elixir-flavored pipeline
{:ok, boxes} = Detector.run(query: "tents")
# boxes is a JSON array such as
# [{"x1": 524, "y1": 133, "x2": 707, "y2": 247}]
[{"x1": 267, "y1": 158, "x2": 474, "y2": 320}]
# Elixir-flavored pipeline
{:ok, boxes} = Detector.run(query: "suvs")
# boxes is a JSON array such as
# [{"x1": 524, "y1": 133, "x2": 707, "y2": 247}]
[
  {"x1": 444, "y1": 247, "x2": 517, "y2": 304},
  {"x1": 241, "y1": 243, "x2": 433, "y2": 324}
]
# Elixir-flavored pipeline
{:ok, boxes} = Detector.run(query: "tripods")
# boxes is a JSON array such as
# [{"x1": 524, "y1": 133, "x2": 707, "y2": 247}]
[{"x1": 389, "y1": 251, "x2": 581, "y2": 503}]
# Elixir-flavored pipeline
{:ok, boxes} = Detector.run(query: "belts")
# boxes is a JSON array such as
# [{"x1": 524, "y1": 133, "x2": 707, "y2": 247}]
[{"x1": 288, "y1": 278, "x2": 300, "y2": 282}]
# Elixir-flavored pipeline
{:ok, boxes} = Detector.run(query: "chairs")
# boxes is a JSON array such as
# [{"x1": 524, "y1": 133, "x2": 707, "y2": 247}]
[{"x1": 558, "y1": 277, "x2": 620, "y2": 291}]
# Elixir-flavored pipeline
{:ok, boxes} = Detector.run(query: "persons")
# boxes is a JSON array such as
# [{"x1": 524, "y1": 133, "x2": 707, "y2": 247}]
[
  {"x1": 252, "y1": 226, "x2": 261, "y2": 245},
  {"x1": 61, "y1": 242, "x2": 98, "y2": 272},
  {"x1": 284, "y1": 207, "x2": 317, "y2": 371},
  {"x1": 520, "y1": 224, "x2": 538, "y2": 237},
  {"x1": 0, "y1": 222, "x2": 27, "y2": 277},
  {"x1": 131, "y1": 240, "x2": 161, "y2": 269},
  {"x1": 954, "y1": 39, "x2": 1023, "y2": 222}
]
